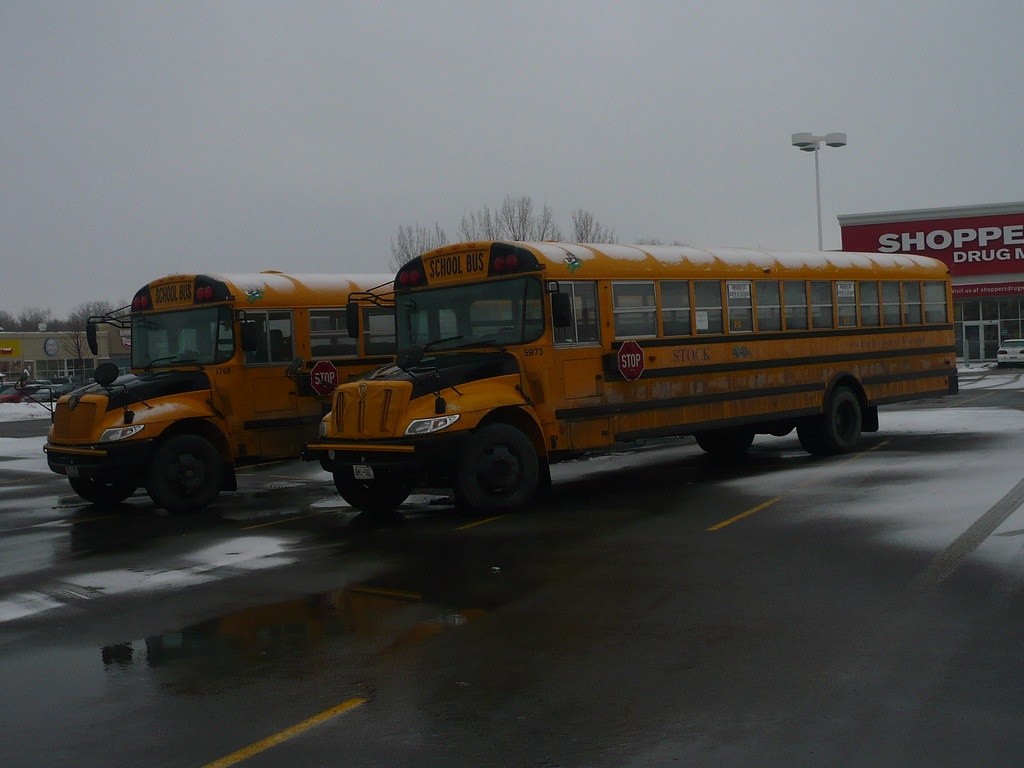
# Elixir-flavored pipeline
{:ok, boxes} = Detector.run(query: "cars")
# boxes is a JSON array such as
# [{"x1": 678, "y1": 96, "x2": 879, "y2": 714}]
[
  {"x1": 996, "y1": 338, "x2": 1024, "y2": 369},
  {"x1": 0, "y1": 375, "x2": 78, "y2": 402}
]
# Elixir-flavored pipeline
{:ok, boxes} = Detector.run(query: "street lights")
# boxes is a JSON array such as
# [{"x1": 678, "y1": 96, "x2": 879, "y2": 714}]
[{"x1": 791, "y1": 132, "x2": 848, "y2": 250}]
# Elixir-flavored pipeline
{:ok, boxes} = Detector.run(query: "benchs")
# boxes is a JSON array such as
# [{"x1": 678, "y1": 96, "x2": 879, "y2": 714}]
[
  {"x1": 312, "y1": 342, "x2": 395, "y2": 357},
  {"x1": 663, "y1": 310, "x2": 947, "y2": 336}
]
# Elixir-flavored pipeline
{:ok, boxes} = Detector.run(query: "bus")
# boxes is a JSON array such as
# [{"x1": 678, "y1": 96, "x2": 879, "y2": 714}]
[
  {"x1": 8, "y1": 267, "x2": 659, "y2": 517},
  {"x1": 287, "y1": 237, "x2": 959, "y2": 506}
]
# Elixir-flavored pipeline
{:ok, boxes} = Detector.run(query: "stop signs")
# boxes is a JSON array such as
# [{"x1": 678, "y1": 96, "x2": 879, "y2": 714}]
[
  {"x1": 311, "y1": 360, "x2": 340, "y2": 396},
  {"x1": 618, "y1": 340, "x2": 646, "y2": 382}
]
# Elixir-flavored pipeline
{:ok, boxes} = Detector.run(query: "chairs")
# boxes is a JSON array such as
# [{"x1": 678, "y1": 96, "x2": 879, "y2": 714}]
[{"x1": 254, "y1": 330, "x2": 286, "y2": 363}]
